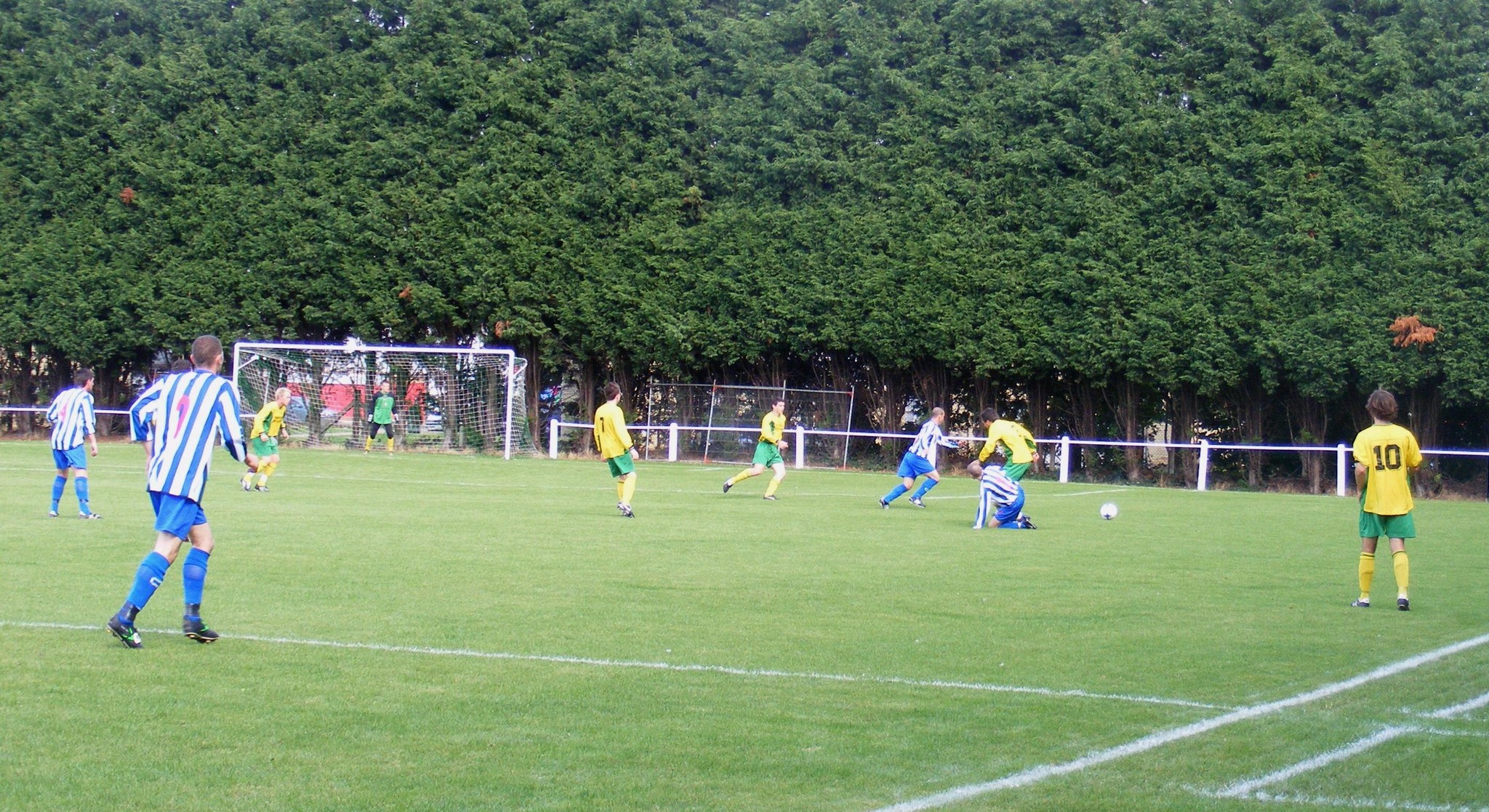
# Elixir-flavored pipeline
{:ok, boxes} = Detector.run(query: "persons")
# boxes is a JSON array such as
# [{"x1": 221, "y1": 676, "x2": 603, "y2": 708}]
[
  {"x1": 363, "y1": 380, "x2": 400, "y2": 456},
  {"x1": 968, "y1": 463, "x2": 1036, "y2": 531},
  {"x1": 593, "y1": 382, "x2": 639, "y2": 518},
  {"x1": 1353, "y1": 389, "x2": 1424, "y2": 609},
  {"x1": 722, "y1": 397, "x2": 788, "y2": 500},
  {"x1": 46, "y1": 369, "x2": 104, "y2": 521},
  {"x1": 239, "y1": 387, "x2": 292, "y2": 493},
  {"x1": 106, "y1": 335, "x2": 259, "y2": 649},
  {"x1": 970, "y1": 407, "x2": 1039, "y2": 485},
  {"x1": 878, "y1": 407, "x2": 966, "y2": 512}
]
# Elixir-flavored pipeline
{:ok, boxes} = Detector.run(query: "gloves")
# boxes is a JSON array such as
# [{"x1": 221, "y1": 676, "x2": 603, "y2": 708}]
[
  {"x1": 367, "y1": 414, "x2": 374, "y2": 424},
  {"x1": 394, "y1": 413, "x2": 399, "y2": 424}
]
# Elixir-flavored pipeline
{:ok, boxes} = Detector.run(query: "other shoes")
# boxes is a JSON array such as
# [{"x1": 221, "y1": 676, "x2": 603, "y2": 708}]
[
  {"x1": 363, "y1": 449, "x2": 369, "y2": 456},
  {"x1": 389, "y1": 451, "x2": 394, "y2": 457}
]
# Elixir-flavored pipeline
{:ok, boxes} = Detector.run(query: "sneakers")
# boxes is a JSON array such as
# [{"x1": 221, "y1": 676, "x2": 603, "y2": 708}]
[
  {"x1": 254, "y1": 483, "x2": 269, "y2": 492},
  {"x1": 1017, "y1": 514, "x2": 1030, "y2": 522},
  {"x1": 879, "y1": 499, "x2": 889, "y2": 509},
  {"x1": 49, "y1": 510, "x2": 60, "y2": 518},
  {"x1": 909, "y1": 497, "x2": 926, "y2": 508},
  {"x1": 106, "y1": 613, "x2": 144, "y2": 649},
  {"x1": 1021, "y1": 519, "x2": 1036, "y2": 530},
  {"x1": 183, "y1": 615, "x2": 219, "y2": 643},
  {"x1": 1351, "y1": 597, "x2": 1370, "y2": 607},
  {"x1": 764, "y1": 494, "x2": 777, "y2": 500},
  {"x1": 239, "y1": 477, "x2": 252, "y2": 492},
  {"x1": 617, "y1": 501, "x2": 635, "y2": 518},
  {"x1": 724, "y1": 477, "x2": 734, "y2": 493},
  {"x1": 1397, "y1": 596, "x2": 1409, "y2": 611},
  {"x1": 79, "y1": 511, "x2": 102, "y2": 519}
]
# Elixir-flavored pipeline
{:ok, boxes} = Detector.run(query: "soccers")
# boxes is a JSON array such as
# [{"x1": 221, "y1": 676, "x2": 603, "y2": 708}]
[{"x1": 1100, "y1": 502, "x2": 1119, "y2": 520}]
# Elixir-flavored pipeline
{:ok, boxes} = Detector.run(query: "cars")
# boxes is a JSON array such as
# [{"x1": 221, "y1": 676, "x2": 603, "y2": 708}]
[{"x1": 290, "y1": 396, "x2": 338, "y2": 422}]
